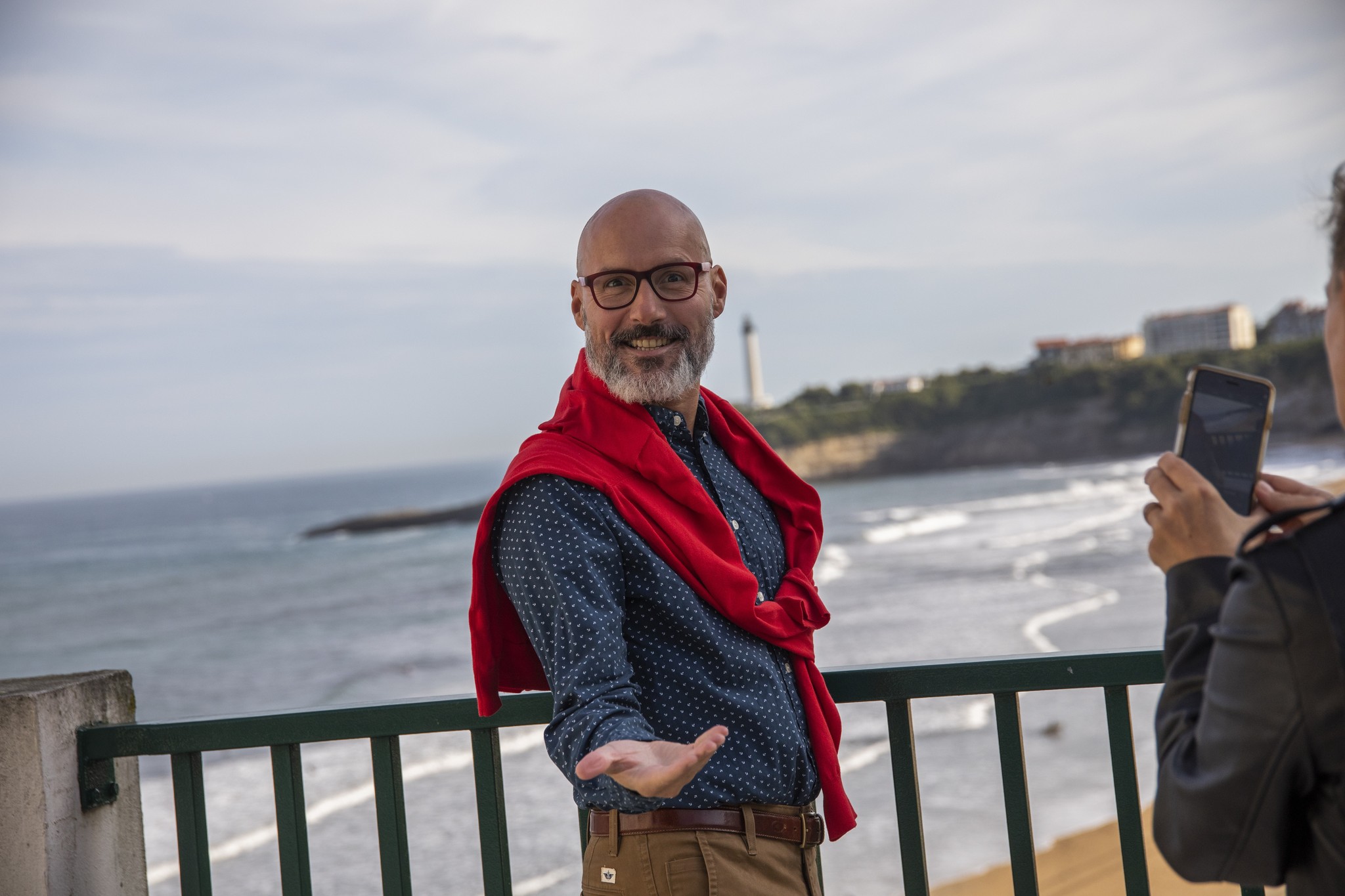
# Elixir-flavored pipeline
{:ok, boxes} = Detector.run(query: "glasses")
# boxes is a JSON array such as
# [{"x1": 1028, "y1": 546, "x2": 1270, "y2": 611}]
[{"x1": 576, "y1": 261, "x2": 711, "y2": 310}]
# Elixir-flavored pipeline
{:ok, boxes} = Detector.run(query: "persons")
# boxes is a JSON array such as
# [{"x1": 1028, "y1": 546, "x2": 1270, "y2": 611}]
[
  {"x1": 494, "y1": 191, "x2": 826, "y2": 896},
  {"x1": 1140, "y1": 155, "x2": 1345, "y2": 896}
]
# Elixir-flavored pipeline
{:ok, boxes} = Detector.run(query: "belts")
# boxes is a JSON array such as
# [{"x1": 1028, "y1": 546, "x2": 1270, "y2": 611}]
[{"x1": 588, "y1": 808, "x2": 826, "y2": 848}]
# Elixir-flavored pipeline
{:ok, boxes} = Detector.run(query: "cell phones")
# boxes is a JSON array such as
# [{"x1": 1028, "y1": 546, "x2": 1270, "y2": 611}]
[{"x1": 1171, "y1": 363, "x2": 1276, "y2": 516}]
